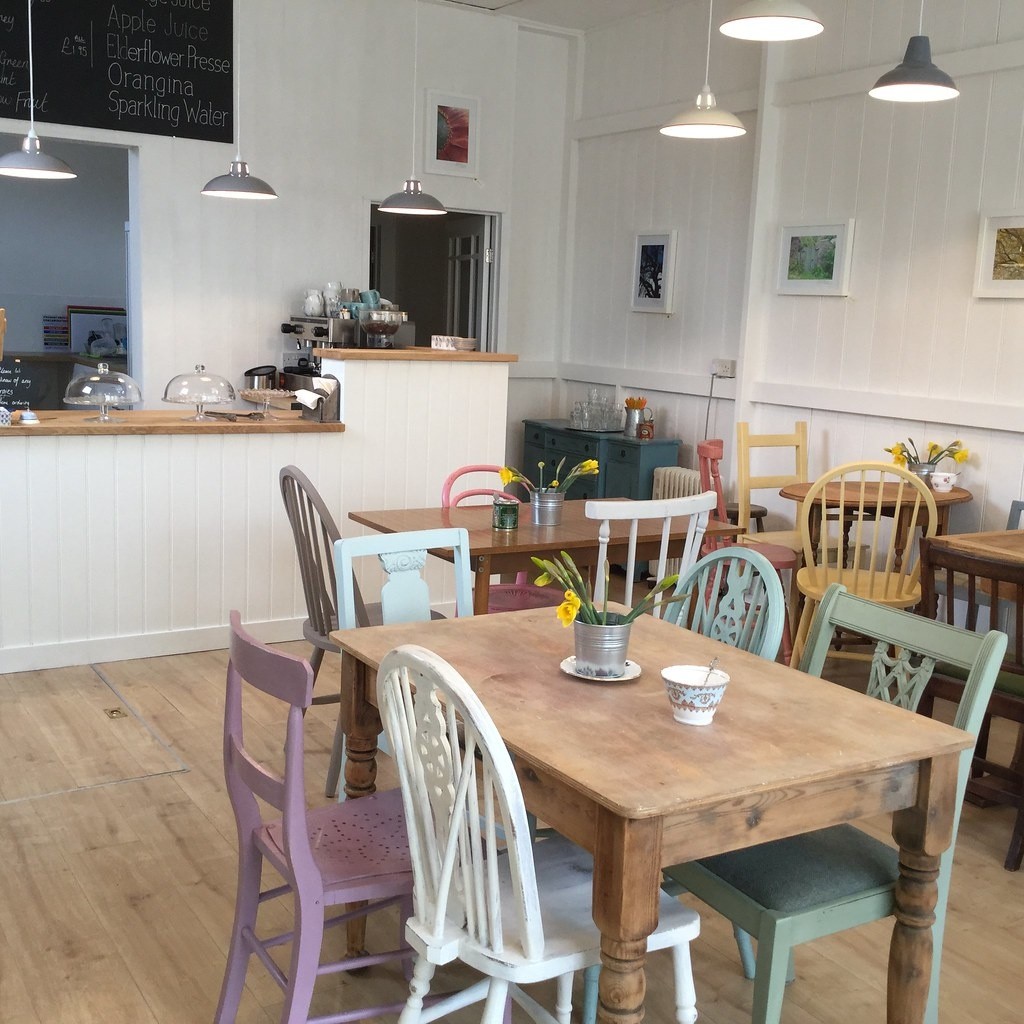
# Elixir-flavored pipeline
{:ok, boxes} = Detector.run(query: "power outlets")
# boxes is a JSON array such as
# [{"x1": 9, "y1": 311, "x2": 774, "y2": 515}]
[{"x1": 716, "y1": 360, "x2": 736, "y2": 378}]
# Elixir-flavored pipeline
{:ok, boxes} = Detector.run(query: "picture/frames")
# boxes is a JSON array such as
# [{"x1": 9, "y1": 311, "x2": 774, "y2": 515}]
[
  {"x1": 971, "y1": 208, "x2": 1024, "y2": 299},
  {"x1": 774, "y1": 218, "x2": 854, "y2": 298},
  {"x1": 423, "y1": 87, "x2": 481, "y2": 178},
  {"x1": 630, "y1": 229, "x2": 678, "y2": 314}
]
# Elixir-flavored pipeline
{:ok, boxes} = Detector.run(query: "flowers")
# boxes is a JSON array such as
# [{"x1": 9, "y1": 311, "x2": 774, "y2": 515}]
[
  {"x1": 884, "y1": 438, "x2": 970, "y2": 467},
  {"x1": 498, "y1": 457, "x2": 598, "y2": 495},
  {"x1": 530, "y1": 548, "x2": 692, "y2": 627}
]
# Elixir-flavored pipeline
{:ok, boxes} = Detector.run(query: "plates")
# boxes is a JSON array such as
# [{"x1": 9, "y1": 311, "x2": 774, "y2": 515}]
[
  {"x1": 453, "y1": 337, "x2": 476, "y2": 350},
  {"x1": 559, "y1": 655, "x2": 642, "y2": 682}
]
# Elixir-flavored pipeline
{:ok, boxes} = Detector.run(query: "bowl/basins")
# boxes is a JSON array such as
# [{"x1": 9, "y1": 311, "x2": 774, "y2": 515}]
[
  {"x1": 83, "y1": 343, "x2": 91, "y2": 354},
  {"x1": 91, "y1": 337, "x2": 117, "y2": 354},
  {"x1": 359, "y1": 309, "x2": 402, "y2": 335}
]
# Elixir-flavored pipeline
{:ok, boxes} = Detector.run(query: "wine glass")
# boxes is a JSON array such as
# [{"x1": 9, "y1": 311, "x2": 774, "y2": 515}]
[
  {"x1": 101, "y1": 318, "x2": 117, "y2": 355},
  {"x1": 113, "y1": 323, "x2": 127, "y2": 354}
]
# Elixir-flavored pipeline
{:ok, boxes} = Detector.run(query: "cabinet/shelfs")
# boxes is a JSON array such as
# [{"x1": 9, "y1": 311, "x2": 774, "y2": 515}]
[{"x1": 519, "y1": 417, "x2": 682, "y2": 583}]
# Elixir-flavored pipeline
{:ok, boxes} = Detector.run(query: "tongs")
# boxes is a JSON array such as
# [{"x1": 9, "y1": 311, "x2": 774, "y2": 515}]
[{"x1": 204, "y1": 411, "x2": 264, "y2": 423}]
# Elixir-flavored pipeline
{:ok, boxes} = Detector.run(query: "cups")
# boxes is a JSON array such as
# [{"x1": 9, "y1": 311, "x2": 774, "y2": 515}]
[
  {"x1": 88, "y1": 329, "x2": 103, "y2": 346},
  {"x1": 302, "y1": 282, "x2": 408, "y2": 321},
  {"x1": 492, "y1": 498, "x2": 518, "y2": 529},
  {"x1": 569, "y1": 386, "x2": 624, "y2": 430},
  {"x1": 930, "y1": 472, "x2": 958, "y2": 493},
  {"x1": 661, "y1": 665, "x2": 729, "y2": 725},
  {"x1": 636, "y1": 419, "x2": 654, "y2": 439}
]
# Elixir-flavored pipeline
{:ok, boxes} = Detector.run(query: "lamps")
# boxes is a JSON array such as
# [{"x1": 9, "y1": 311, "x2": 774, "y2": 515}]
[
  {"x1": 377, "y1": 0, "x2": 447, "y2": 214},
  {"x1": 718, "y1": 0, "x2": 824, "y2": 41},
  {"x1": 0, "y1": 0, "x2": 76, "y2": 179},
  {"x1": 658, "y1": 0, "x2": 746, "y2": 138},
  {"x1": 200, "y1": 0, "x2": 278, "y2": 199},
  {"x1": 868, "y1": 0, "x2": 959, "y2": 103}
]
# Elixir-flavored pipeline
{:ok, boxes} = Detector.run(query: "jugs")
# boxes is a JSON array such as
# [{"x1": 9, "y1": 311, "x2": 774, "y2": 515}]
[{"x1": 624, "y1": 406, "x2": 653, "y2": 436}]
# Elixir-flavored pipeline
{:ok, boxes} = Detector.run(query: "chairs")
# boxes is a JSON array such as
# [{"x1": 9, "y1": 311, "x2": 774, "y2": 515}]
[{"x1": 208, "y1": 422, "x2": 1023, "y2": 1024}]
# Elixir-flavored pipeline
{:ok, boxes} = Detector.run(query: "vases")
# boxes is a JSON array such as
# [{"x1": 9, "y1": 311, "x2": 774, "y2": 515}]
[
  {"x1": 574, "y1": 610, "x2": 633, "y2": 677},
  {"x1": 530, "y1": 488, "x2": 565, "y2": 526},
  {"x1": 907, "y1": 464, "x2": 936, "y2": 488}
]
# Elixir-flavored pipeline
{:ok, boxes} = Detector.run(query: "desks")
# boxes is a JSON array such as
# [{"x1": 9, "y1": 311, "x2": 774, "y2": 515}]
[
  {"x1": 780, "y1": 481, "x2": 972, "y2": 652},
  {"x1": 346, "y1": 490, "x2": 745, "y2": 618},
  {"x1": 329, "y1": 600, "x2": 977, "y2": 1023},
  {"x1": 927, "y1": 529, "x2": 1024, "y2": 809},
  {"x1": 5, "y1": 351, "x2": 127, "y2": 410}
]
